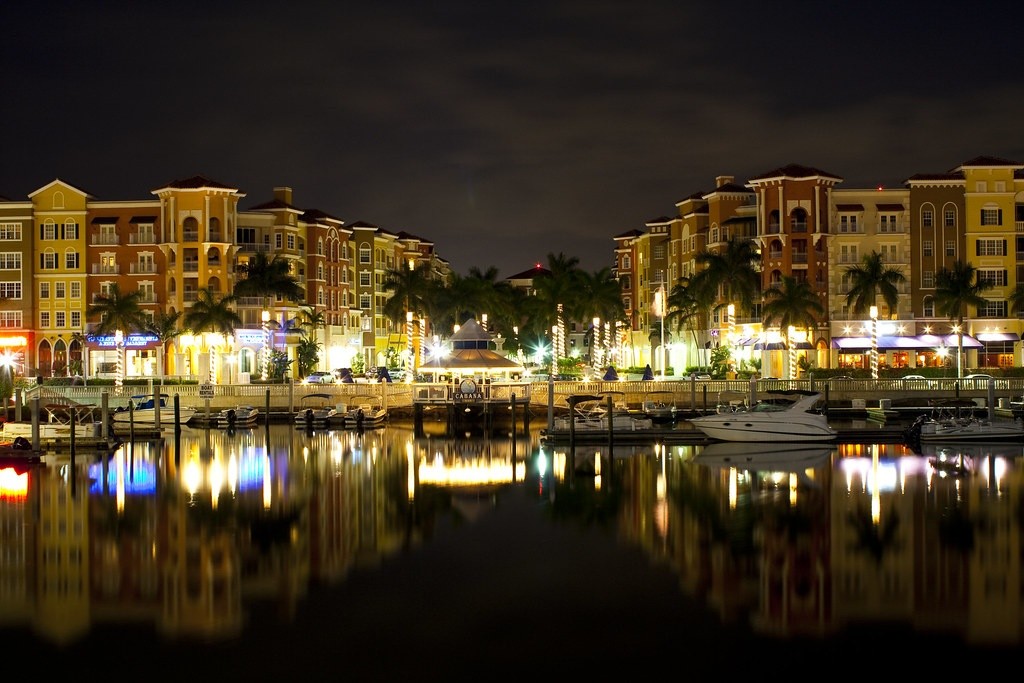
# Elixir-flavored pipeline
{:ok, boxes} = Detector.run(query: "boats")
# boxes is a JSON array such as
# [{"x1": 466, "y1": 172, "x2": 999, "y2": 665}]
[
  {"x1": 541, "y1": 393, "x2": 839, "y2": 446},
  {"x1": 688, "y1": 443, "x2": 840, "y2": 489},
  {"x1": 293, "y1": 393, "x2": 389, "y2": 430},
  {"x1": 911, "y1": 378, "x2": 1024, "y2": 442},
  {"x1": 0, "y1": 394, "x2": 260, "y2": 450}
]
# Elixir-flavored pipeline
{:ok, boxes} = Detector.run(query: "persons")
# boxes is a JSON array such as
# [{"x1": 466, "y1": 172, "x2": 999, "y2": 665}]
[
  {"x1": 643, "y1": 365, "x2": 653, "y2": 380},
  {"x1": 340, "y1": 368, "x2": 353, "y2": 382},
  {"x1": 603, "y1": 366, "x2": 617, "y2": 381}
]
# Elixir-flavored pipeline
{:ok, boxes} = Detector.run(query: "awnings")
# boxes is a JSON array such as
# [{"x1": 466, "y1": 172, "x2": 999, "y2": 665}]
[
  {"x1": 754, "y1": 330, "x2": 816, "y2": 350},
  {"x1": 975, "y1": 333, "x2": 1020, "y2": 368},
  {"x1": 917, "y1": 334, "x2": 984, "y2": 369},
  {"x1": 734, "y1": 337, "x2": 761, "y2": 350},
  {"x1": 832, "y1": 337, "x2": 934, "y2": 370}
]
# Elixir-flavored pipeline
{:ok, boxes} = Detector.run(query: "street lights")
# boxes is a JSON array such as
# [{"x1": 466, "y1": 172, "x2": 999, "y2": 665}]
[{"x1": 652, "y1": 274, "x2": 665, "y2": 386}]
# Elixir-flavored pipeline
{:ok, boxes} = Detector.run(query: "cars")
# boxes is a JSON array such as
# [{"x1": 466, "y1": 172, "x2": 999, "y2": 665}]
[
  {"x1": 954, "y1": 373, "x2": 994, "y2": 386},
  {"x1": 304, "y1": 370, "x2": 339, "y2": 385},
  {"x1": 366, "y1": 367, "x2": 405, "y2": 378},
  {"x1": 889, "y1": 374, "x2": 932, "y2": 393}
]
